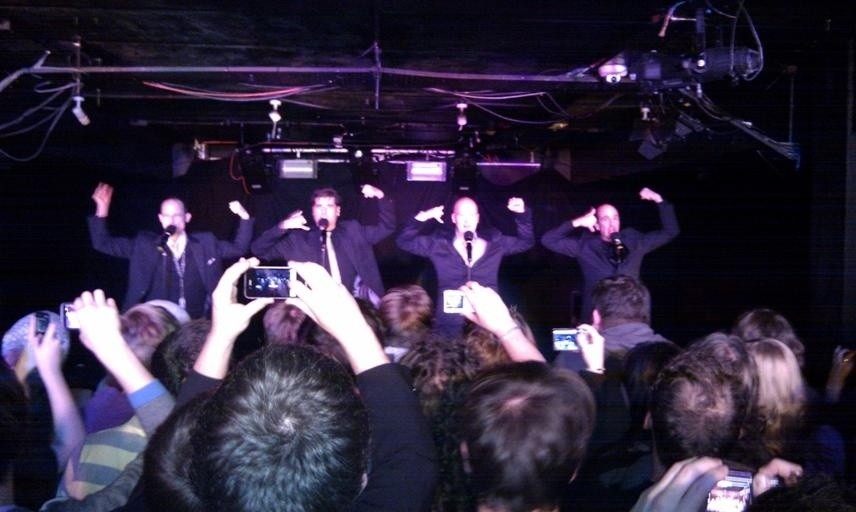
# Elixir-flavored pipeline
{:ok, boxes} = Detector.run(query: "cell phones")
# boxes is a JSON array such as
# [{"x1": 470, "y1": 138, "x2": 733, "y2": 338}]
[
  {"x1": 702, "y1": 461, "x2": 781, "y2": 512},
  {"x1": 442, "y1": 289, "x2": 474, "y2": 313},
  {"x1": 60, "y1": 303, "x2": 79, "y2": 330},
  {"x1": 243, "y1": 267, "x2": 298, "y2": 299},
  {"x1": 552, "y1": 328, "x2": 579, "y2": 352},
  {"x1": 35, "y1": 311, "x2": 50, "y2": 333}
]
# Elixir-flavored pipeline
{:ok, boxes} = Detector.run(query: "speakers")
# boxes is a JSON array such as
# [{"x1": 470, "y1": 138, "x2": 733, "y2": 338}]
[
  {"x1": 453, "y1": 167, "x2": 475, "y2": 195},
  {"x1": 241, "y1": 152, "x2": 272, "y2": 194}
]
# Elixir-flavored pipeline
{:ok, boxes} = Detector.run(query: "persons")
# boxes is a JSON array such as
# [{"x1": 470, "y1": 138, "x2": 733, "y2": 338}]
[
  {"x1": 394, "y1": 196, "x2": 534, "y2": 338},
  {"x1": 0, "y1": 258, "x2": 856, "y2": 512},
  {"x1": 85, "y1": 178, "x2": 255, "y2": 323},
  {"x1": 540, "y1": 186, "x2": 680, "y2": 332},
  {"x1": 252, "y1": 182, "x2": 395, "y2": 307}
]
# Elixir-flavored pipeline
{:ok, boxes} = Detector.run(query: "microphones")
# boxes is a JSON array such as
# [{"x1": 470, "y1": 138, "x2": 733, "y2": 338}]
[
  {"x1": 610, "y1": 231, "x2": 624, "y2": 252},
  {"x1": 157, "y1": 225, "x2": 175, "y2": 252},
  {"x1": 464, "y1": 231, "x2": 473, "y2": 260},
  {"x1": 318, "y1": 218, "x2": 329, "y2": 251}
]
